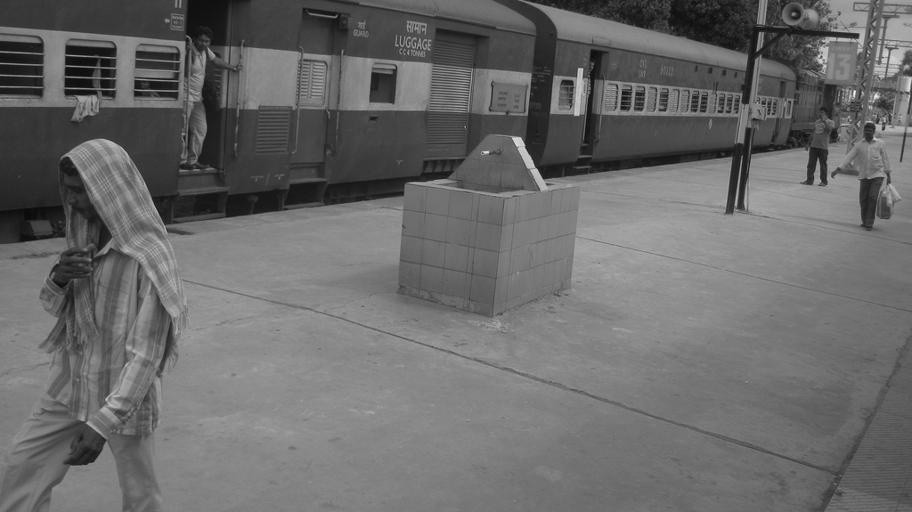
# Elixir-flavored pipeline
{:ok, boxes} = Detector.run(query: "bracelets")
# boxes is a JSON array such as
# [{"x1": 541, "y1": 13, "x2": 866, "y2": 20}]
[{"x1": 233, "y1": 65, "x2": 237, "y2": 72}]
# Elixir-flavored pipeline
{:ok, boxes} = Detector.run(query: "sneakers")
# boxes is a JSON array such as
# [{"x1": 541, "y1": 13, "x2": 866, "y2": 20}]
[
  {"x1": 178, "y1": 162, "x2": 208, "y2": 172},
  {"x1": 860, "y1": 223, "x2": 873, "y2": 230},
  {"x1": 799, "y1": 180, "x2": 814, "y2": 185},
  {"x1": 818, "y1": 181, "x2": 828, "y2": 186}
]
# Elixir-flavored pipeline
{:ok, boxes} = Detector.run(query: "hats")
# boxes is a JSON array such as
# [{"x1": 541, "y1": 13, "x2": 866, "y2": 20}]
[{"x1": 876, "y1": 183, "x2": 902, "y2": 219}]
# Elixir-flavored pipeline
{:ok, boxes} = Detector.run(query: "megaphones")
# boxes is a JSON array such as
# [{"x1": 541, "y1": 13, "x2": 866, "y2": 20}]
[
  {"x1": 799, "y1": 9, "x2": 819, "y2": 30},
  {"x1": 782, "y1": 2, "x2": 805, "y2": 25}
]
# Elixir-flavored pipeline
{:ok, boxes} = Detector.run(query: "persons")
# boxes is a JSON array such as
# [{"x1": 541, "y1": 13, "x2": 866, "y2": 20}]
[
  {"x1": 179, "y1": 24, "x2": 246, "y2": 172},
  {"x1": 0, "y1": 137, "x2": 189, "y2": 511},
  {"x1": 830, "y1": 122, "x2": 891, "y2": 231},
  {"x1": 581, "y1": 58, "x2": 597, "y2": 140},
  {"x1": 798, "y1": 106, "x2": 837, "y2": 186},
  {"x1": 874, "y1": 111, "x2": 896, "y2": 132}
]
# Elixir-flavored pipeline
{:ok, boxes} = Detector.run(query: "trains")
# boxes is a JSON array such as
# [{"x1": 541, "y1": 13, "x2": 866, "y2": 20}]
[{"x1": 1, "y1": 0, "x2": 842, "y2": 247}]
